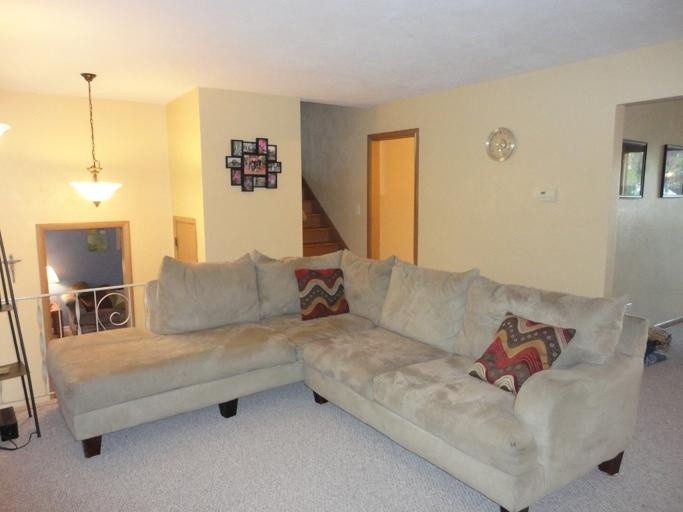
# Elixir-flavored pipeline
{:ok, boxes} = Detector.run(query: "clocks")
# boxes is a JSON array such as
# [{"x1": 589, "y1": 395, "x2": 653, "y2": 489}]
[{"x1": 484, "y1": 125, "x2": 518, "y2": 164}]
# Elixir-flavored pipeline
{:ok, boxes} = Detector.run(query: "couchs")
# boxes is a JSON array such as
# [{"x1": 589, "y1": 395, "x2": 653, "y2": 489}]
[
  {"x1": 45, "y1": 248, "x2": 650, "y2": 510},
  {"x1": 59, "y1": 282, "x2": 130, "y2": 336}
]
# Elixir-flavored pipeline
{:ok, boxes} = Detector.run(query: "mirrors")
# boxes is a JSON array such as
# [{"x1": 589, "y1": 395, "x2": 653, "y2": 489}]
[
  {"x1": 618, "y1": 139, "x2": 648, "y2": 199},
  {"x1": 658, "y1": 144, "x2": 683, "y2": 199}
]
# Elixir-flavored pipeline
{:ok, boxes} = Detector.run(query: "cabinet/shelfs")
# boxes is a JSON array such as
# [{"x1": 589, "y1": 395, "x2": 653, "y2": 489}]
[{"x1": 0, "y1": 228, "x2": 41, "y2": 438}]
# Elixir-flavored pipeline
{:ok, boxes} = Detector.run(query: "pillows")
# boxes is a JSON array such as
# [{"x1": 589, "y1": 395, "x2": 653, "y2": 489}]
[
  {"x1": 466, "y1": 310, "x2": 577, "y2": 397},
  {"x1": 292, "y1": 267, "x2": 351, "y2": 321},
  {"x1": 66, "y1": 285, "x2": 118, "y2": 318}
]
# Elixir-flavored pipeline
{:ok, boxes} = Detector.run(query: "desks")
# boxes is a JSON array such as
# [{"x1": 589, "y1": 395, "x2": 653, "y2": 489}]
[{"x1": 49, "y1": 303, "x2": 64, "y2": 338}]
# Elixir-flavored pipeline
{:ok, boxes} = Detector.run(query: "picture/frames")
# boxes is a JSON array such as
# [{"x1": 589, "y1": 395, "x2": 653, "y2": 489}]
[{"x1": 224, "y1": 137, "x2": 282, "y2": 193}]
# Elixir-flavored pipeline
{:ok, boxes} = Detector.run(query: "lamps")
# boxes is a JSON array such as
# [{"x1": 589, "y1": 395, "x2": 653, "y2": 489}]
[
  {"x1": 47, "y1": 267, "x2": 58, "y2": 293},
  {"x1": 67, "y1": 73, "x2": 122, "y2": 209}
]
